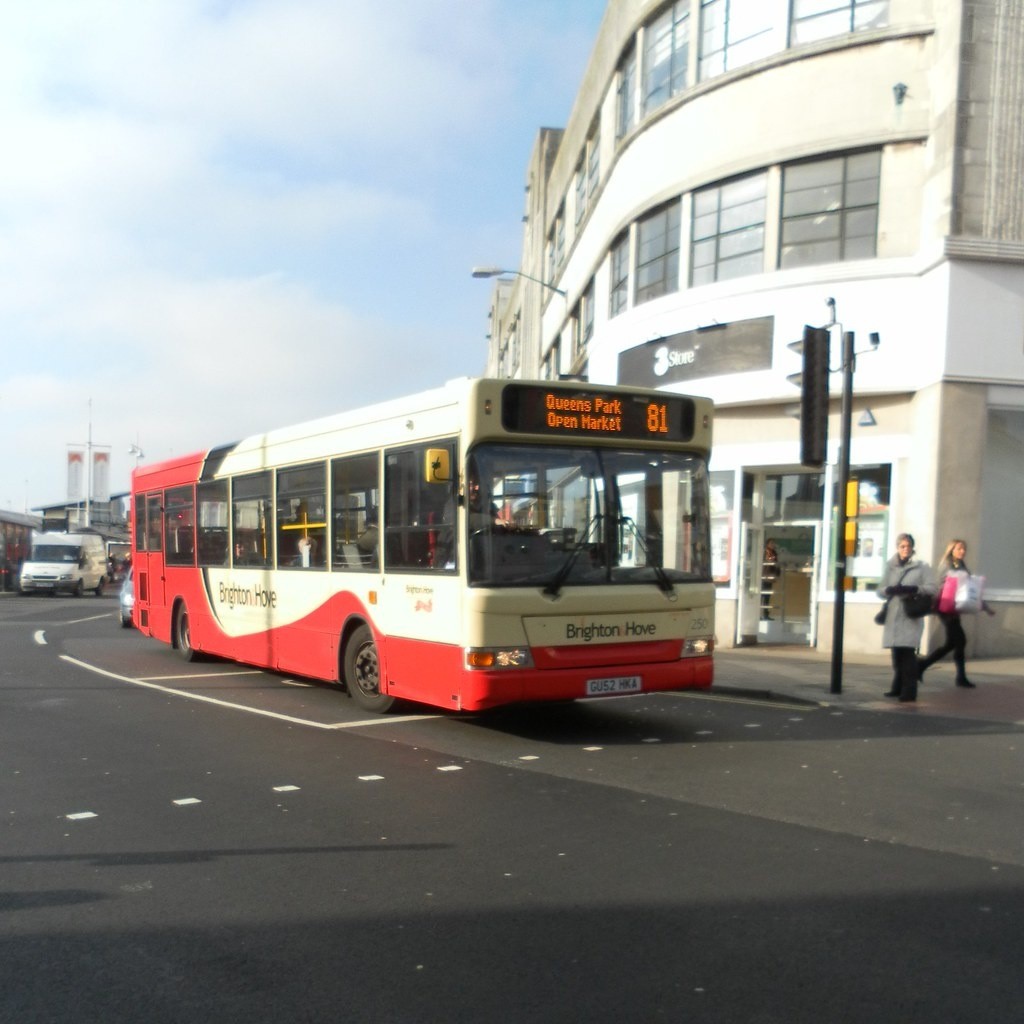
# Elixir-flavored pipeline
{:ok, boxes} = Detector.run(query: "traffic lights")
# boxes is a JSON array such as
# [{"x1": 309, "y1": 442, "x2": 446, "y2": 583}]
[{"x1": 785, "y1": 326, "x2": 831, "y2": 468}]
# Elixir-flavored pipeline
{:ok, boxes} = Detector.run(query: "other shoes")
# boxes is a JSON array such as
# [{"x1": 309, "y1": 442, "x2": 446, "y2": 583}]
[
  {"x1": 898, "y1": 694, "x2": 917, "y2": 702},
  {"x1": 955, "y1": 678, "x2": 975, "y2": 687},
  {"x1": 884, "y1": 691, "x2": 900, "y2": 696},
  {"x1": 760, "y1": 615, "x2": 774, "y2": 620},
  {"x1": 916, "y1": 658, "x2": 925, "y2": 682}
]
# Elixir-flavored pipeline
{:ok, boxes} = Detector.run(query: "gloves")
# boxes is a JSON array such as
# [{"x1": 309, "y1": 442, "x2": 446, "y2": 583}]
[{"x1": 886, "y1": 583, "x2": 917, "y2": 594}]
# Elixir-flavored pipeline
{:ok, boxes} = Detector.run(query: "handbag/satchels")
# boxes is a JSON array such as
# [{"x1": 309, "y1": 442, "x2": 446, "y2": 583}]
[
  {"x1": 874, "y1": 604, "x2": 887, "y2": 624},
  {"x1": 939, "y1": 573, "x2": 959, "y2": 612},
  {"x1": 954, "y1": 573, "x2": 985, "y2": 611},
  {"x1": 902, "y1": 593, "x2": 933, "y2": 618}
]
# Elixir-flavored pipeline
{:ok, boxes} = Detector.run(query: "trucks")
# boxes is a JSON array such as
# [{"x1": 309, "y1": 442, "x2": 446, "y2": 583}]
[{"x1": 18, "y1": 531, "x2": 107, "y2": 598}]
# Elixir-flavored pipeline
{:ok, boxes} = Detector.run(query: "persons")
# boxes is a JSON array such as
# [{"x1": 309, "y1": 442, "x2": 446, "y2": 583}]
[
  {"x1": 435, "y1": 470, "x2": 525, "y2": 570},
  {"x1": 118, "y1": 552, "x2": 132, "y2": 573},
  {"x1": 223, "y1": 536, "x2": 257, "y2": 566},
  {"x1": 761, "y1": 537, "x2": 781, "y2": 620},
  {"x1": 289, "y1": 536, "x2": 322, "y2": 567},
  {"x1": 6, "y1": 544, "x2": 22, "y2": 590},
  {"x1": 917, "y1": 539, "x2": 996, "y2": 688},
  {"x1": 142, "y1": 514, "x2": 161, "y2": 549},
  {"x1": 285, "y1": 505, "x2": 302, "y2": 553},
  {"x1": 387, "y1": 451, "x2": 418, "y2": 564},
  {"x1": 158, "y1": 513, "x2": 193, "y2": 553},
  {"x1": 107, "y1": 553, "x2": 118, "y2": 583},
  {"x1": 877, "y1": 533, "x2": 938, "y2": 702}
]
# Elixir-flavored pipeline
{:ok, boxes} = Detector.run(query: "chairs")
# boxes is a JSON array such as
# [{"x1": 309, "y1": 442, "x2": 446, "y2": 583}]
[
  {"x1": 427, "y1": 510, "x2": 442, "y2": 570},
  {"x1": 138, "y1": 522, "x2": 269, "y2": 564}
]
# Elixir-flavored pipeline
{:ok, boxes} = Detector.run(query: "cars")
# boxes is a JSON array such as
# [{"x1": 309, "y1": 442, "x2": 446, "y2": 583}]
[
  {"x1": 119, "y1": 566, "x2": 134, "y2": 628},
  {"x1": 131, "y1": 377, "x2": 715, "y2": 715}
]
